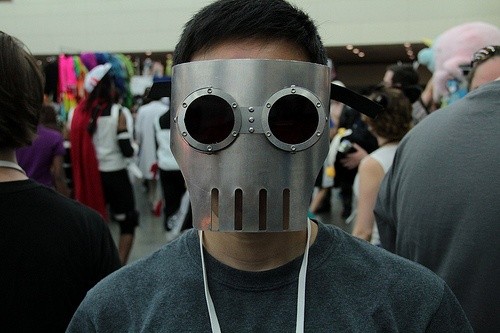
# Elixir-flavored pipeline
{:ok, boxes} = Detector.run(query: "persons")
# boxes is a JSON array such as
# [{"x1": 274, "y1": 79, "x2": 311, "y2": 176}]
[
  {"x1": 373, "y1": 76, "x2": 500, "y2": 333},
  {"x1": 382, "y1": 64, "x2": 423, "y2": 105},
  {"x1": 352, "y1": 88, "x2": 414, "y2": 247},
  {"x1": 0, "y1": 31, "x2": 122, "y2": 333},
  {"x1": 307, "y1": 80, "x2": 379, "y2": 224},
  {"x1": 134, "y1": 76, "x2": 194, "y2": 243},
  {"x1": 65, "y1": 0, "x2": 473, "y2": 333},
  {"x1": 67, "y1": 62, "x2": 144, "y2": 266},
  {"x1": 15, "y1": 125, "x2": 66, "y2": 192}
]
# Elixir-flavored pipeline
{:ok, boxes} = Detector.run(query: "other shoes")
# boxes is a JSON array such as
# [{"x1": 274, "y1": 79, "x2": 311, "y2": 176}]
[
  {"x1": 151, "y1": 199, "x2": 161, "y2": 217},
  {"x1": 342, "y1": 209, "x2": 351, "y2": 218},
  {"x1": 315, "y1": 205, "x2": 330, "y2": 213}
]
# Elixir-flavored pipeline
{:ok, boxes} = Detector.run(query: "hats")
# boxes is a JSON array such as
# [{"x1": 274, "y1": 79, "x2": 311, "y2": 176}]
[{"x1": 84, "y1": 64, "x2": 112, "y2": 93}]
